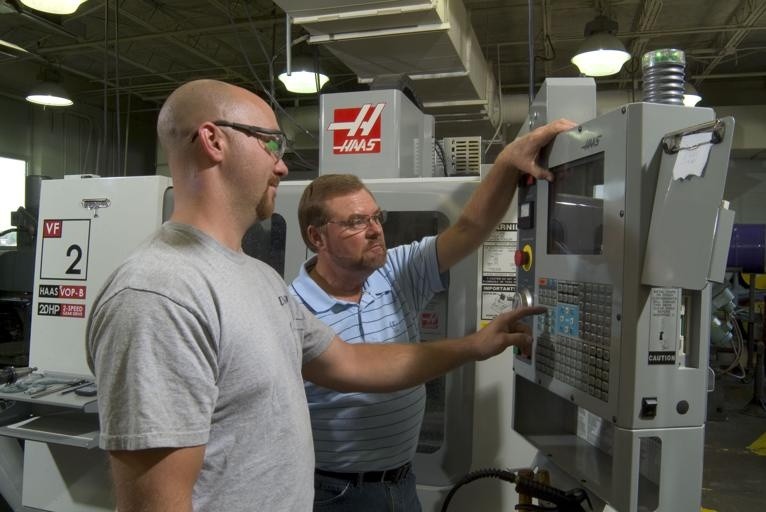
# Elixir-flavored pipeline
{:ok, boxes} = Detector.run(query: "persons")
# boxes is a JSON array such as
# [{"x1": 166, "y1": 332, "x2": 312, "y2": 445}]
[
  {"x1": 280, "y1": 118, "x2": 579, "y2": 512},
  {"x1": 82, "y1": 78, "x2": 551, "y2": 512}
]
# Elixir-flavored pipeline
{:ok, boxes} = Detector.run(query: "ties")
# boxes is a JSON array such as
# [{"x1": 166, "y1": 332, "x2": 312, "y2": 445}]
[{"x1": 546, "y1": 151, "x2": 606, "y2": 257}]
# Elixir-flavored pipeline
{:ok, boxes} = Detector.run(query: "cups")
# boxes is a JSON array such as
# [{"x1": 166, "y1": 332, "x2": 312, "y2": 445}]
[
  {"x1": 188, "y1": 120, "x2": 287, "y2": 161},
  {"x1": 315, "y1": 209, "x2": 390, "y2": 232}
]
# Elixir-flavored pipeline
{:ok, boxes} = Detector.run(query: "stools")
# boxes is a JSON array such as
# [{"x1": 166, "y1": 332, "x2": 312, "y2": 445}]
[{"x1": 308, "y1": 459, "x2": 414, "y2": 486}]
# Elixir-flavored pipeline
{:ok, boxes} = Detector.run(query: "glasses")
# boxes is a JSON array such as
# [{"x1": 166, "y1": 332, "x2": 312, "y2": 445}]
[
  {"x1": 277, "y1": 39, "x2": 331, "y2": 94},
  {"x1": 572, "y1": 13, "x2": 631, "y2": 77},
  {"x1": 19, "y1": 0, "x2": 89, "y2": 18},
  {"x1": 26, "y1": 60, "x2": 75, "y2": 110},
  {"x1": 683, "y1": 81, "x2": 702, "y2": 108}
]
